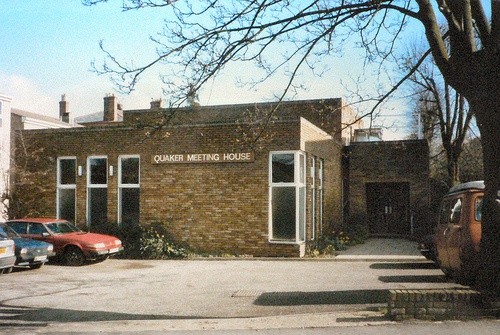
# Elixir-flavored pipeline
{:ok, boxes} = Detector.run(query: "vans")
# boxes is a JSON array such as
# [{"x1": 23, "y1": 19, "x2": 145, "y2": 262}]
[{"x1": 436, "y1": 180, "x2": 484, "y2": 283}]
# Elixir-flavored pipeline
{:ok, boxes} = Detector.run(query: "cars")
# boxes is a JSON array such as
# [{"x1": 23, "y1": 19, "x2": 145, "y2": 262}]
[
  {"x1": 419, "y1": 233, "x2": 438, "y2": 259},
  {"x1": 0, "y1": 235, "x2": 15, "y2": 271},
  {"x1": 0, "y1": 224, "x2": 56, "y2": 270},
  {"x1": 5, "y1": 217, "x2": 125, "y2": 265}
]
